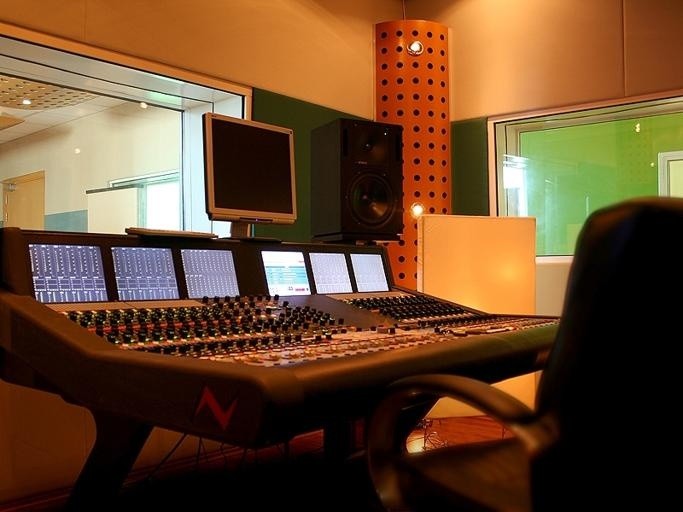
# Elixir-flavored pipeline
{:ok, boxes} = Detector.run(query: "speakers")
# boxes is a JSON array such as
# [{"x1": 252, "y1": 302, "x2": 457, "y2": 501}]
[{"x1": 311, "y1": 117, "x2": 404, "y2": 245}]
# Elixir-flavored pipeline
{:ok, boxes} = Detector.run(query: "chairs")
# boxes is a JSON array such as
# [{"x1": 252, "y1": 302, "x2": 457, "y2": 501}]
[{"x1": 347, "y1": 197, "x2": 682, "y2": 512}]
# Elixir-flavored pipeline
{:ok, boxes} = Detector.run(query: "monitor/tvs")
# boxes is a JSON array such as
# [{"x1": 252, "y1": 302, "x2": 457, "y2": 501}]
[
  {"x1": 28, "y1": 244, "x2": 108, "y2": 303},
  {"x1": 111, "y1": 247, "x2": 179, "y2": 300},
  {"x1": 202, "y1": 109, "x2": 298, "y2": 239},
  {"x1": 309, "y1": 253, "x2": 353, "y2": 294},
  {"x1": 261, "y1": 250, "x2": 312, "y2": 296},
  {"x1": 181, "y1": 250, "x2": 240, "y2": 298},
  {"x1": 350, "y1": 254, "x2": 391, "y2": 292}
]
